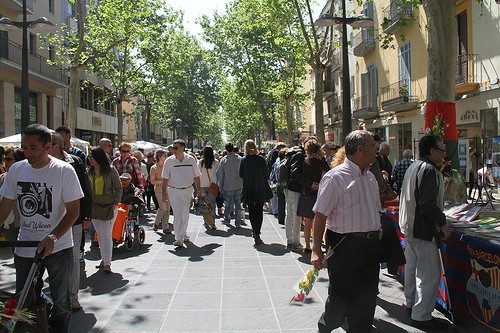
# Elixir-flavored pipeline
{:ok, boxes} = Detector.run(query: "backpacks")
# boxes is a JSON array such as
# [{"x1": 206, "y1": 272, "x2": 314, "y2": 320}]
[{"x1": 279, "y1": 159, "x2": 288, "y2": 187}]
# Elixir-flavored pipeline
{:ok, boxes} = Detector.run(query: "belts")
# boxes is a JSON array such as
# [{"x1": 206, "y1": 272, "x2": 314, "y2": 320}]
[
  {"x1": 168, "y1": 184, "x2": 192, "y2": 189},
  {"x1": 326, "y1": 228, "x2": 384, "y2": 239}
]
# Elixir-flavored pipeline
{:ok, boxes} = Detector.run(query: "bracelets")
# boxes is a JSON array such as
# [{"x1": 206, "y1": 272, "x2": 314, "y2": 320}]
[{"x1": 48, "y1": 233, "x2": 57, "y2": 243}]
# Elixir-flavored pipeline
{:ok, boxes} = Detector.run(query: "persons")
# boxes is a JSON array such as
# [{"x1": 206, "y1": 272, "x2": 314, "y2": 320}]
[
  {"x1": 398, "y1": 136, "x2": 451, "y2": 329},
  {"x1": 133, "y1": 139, "x2": 273, "y2": 246},
  {"x1": 0, "y1": 124, "x2": 85, "y2": 333},
  {"x1": 48, "y1": 131, "x2": 93, "y2": 309},
  {"x1": 268, "y1": 135, "x2": 346, "y2": 254},
  {"x1": 312, "y1": 130, "x2": 383, "y2": 333},
  {"x1": 468, "y1": 167, "x2": 495, "y2": 201},
  {"x1": 56, "y1": 127, "x2": 149, "y2": 271},
  {"x1": 370, "y1": 142, "x2": 414, "y2": 200},
  {"x1": 0, "y1": 146, "x2": 26, "y2": 250},
  {"x1": 441, "y1": 166, "x2": 466, "y2": 202}
]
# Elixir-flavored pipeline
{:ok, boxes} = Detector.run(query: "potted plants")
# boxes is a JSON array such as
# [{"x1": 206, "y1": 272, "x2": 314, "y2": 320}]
[{"x1": 399, "y1": 87, "x2": 409, "y2": 103}]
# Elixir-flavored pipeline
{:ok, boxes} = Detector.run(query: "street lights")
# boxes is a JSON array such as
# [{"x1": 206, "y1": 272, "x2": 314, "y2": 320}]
[
  {"x1": 314, "y1": 1, "x2": 376, "y2": 139},
  {"x1": 0, "y1": 1, "x2": 57, "y2": 131}
]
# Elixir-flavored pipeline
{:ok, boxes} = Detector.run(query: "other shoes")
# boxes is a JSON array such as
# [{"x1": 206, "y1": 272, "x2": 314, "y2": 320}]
[
  {"x1": 252, "y1": 233, "x2": 262, "y2": 245},
  {"x1": 305, "y1": 249, "x2": 312, "y2": 254},
  {"x1": 287, "y1": 244, "x2": 305, "y2": 251},
  {"x1": 204, "y1": 223, "x2": 216, "y2": 230},
  {"x1": 72, "y1": 299, "x2": 81, "y2": 309},
  {"x1": 46, "y1": 302, "x2": 53, "y2": 318},
  {"x1": 410, "y1": 316, "x2": 451, "y2": 329},
  {"x1": 153, "y1": 223, "x2": 158, "y2": 232},
  {"x1": 221, "y1": 220, "x2": 230, "y2": 224},
  {"x1": 163, "y1": 229, "x2": 171, "y2": 234},
  {"x1": 173, "y1": 240, "x2": 184, "y2": 247},
  {"x1": 103, "y1": 265, "x2": 111, "y2": 272},
  {"x1": 235, "y1": 221, "x2": 241, "y2": 227},
  {"x1": 93, "y1": 241, "x2": 99, "y2": 247},
  {"x1": 79, "y1": 249, "x2": 84, "y2": 262},
  {"x1": 96, "y1": 261, "x2": 105, "y2": 268},
  {"x1": 406, "y1": 308, "x2": 412, "y2": 318},
  {"x1": 184, "y1": 235, "x2": 189, "y2": 241}
]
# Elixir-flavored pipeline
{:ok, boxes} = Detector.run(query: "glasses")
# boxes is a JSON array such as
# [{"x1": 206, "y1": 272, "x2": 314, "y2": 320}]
[
  {"x1": 3, "y1": 158, "x2": 17, "y2": 161},
  {"x1": 172, "y1": 146, "x2": 178, "y2": 150},
  {"x1": 122, "y1": 150, "x2": 130, "y2": 153},
  {"x1": 433, "y1": 146, "x2": 446, "y2": 154}
]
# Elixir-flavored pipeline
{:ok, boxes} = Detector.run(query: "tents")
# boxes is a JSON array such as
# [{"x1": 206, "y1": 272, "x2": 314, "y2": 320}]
[{"x1": 0, "y1": 128, "x2": 90, "y2": 153}]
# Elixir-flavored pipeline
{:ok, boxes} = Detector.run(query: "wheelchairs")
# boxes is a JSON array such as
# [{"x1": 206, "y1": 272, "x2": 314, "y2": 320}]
[{"x1": 93, "y1": 188, "x2": 146, "y2": 252}]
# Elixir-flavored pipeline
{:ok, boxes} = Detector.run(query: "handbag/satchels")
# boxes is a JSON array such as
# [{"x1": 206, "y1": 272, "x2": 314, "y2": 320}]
[{"x1": 208, "y1": 182, "x2": 219, "y2": 195}]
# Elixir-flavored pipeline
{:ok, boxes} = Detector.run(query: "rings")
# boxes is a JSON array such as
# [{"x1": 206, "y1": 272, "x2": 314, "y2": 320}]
[{"x1": 49, "y1": 251, "x2": 51, "y2": 252}]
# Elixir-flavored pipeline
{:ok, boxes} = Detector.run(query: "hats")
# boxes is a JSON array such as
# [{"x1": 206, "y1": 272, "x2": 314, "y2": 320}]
[{"x1": 120, "y1": 173, "x2": 132, "y2": 180}]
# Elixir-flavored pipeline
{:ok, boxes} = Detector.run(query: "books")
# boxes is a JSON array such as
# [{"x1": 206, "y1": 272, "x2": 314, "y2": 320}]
[{"x1": 444, "y1": 199, "x2": 500, "y2": 243}]
